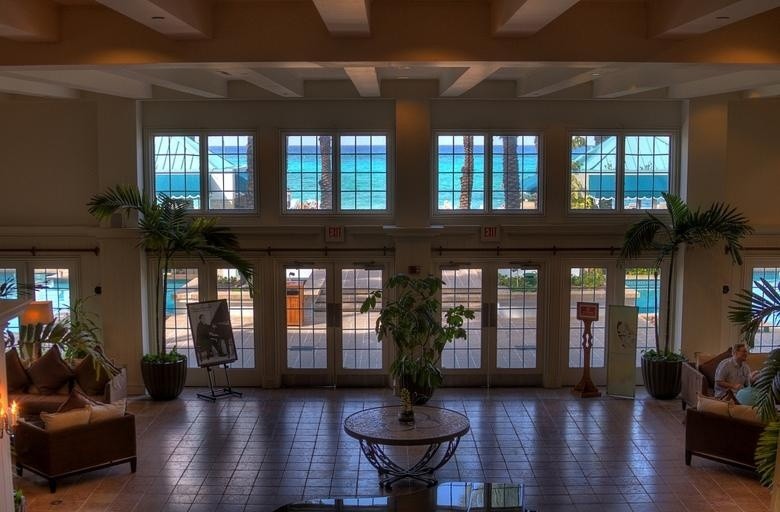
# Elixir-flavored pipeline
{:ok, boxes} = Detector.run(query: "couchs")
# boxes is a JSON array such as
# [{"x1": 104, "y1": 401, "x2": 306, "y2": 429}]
[
  {"x1": 685, "y1": 354, "x2": 776, "y2": 477},
  {"x1": 15, "y1": 411, "x2": 137, "y2": 494},
  {"x1": 6, "y1": 360, "x2": 127, "y2": 418}
]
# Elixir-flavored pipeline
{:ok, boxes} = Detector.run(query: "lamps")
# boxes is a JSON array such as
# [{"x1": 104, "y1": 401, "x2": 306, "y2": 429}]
[{"x1": 22, "y1": 301, "x2": 54, "y2": 324}]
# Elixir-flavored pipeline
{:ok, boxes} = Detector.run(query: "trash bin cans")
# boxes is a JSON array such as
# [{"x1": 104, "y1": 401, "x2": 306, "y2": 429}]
[{"x1": 284, "y1": 278, "x2": 307, "y2": 326}]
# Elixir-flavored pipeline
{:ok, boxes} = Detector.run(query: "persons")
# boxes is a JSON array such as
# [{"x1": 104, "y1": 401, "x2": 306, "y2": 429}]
[
  {"x1": 197, "y1": 314, "x2": 227, "y2": 357},
  {"x1": 714, "y1": 344, "x2": 769, "y2": 406}
]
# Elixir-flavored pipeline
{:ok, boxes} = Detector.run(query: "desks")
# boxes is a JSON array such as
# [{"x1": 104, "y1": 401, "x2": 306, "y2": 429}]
[{"x1": 343, "y1": 405, "x2": 471, "y2": 493}]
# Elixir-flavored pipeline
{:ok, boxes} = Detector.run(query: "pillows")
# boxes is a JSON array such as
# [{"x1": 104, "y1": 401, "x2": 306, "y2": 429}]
[
  {"x1": 4, "y1": 345, "x2": 118, "y2": 396},
  {"x1": 40, "y1": 399, "x2": 127, "y2": 431},
  {"x1": 696, "y1": 347, "x2": 733, "y2": 386}
]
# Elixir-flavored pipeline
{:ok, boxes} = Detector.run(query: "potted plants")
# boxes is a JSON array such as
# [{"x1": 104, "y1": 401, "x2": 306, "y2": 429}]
[
  {"x1": 361, "y1": 273, "x2": 477, "y2": 406},
  {"x1": 608, "y1": 190, "x2": 756, "y2": 400},
  {"x1": 86, "y1": 184, "x2": 257, "y2": 402}
]
